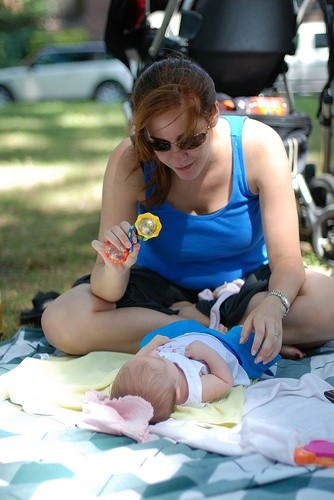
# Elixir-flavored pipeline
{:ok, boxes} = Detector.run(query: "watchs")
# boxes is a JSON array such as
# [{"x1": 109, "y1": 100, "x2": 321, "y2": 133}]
[{"x1": 263, "y1": 289, "x2": 291, "y2": 318}]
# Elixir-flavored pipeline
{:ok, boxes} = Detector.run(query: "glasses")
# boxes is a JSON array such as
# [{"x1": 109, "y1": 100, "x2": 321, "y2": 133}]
[{"x1": 148, "y1": 113, "x2": 216, "y2": 152}]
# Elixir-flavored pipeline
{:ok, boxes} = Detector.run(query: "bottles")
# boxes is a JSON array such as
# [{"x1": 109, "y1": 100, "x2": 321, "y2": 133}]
[{"x1": 223, "y1": 95, "x2": 290, "y2": 115}]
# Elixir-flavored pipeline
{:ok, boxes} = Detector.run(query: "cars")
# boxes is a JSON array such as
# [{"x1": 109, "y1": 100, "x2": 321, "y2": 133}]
[{"x1": 0, "y1": 40, "x2": 134, "y2": 106}]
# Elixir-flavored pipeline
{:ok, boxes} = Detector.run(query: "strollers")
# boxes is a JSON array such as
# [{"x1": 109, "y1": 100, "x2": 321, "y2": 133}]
[{"x1": 103, "y1": 1, "x2": 334, "y2": 267}]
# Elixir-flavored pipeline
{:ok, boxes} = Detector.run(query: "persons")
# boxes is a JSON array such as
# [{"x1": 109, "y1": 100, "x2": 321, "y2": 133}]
[
  {"x1": 40, "y1": 53, "x2": 334, "y2": 368},
  {"x1": 109, "y1": 305, "x2": 279, "y2": 425}
]
